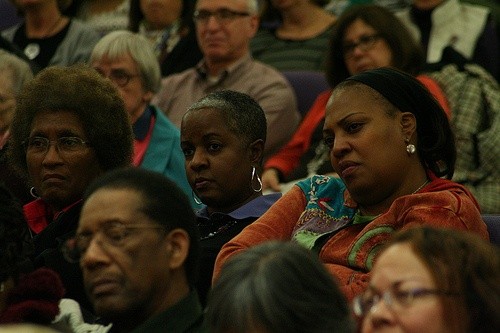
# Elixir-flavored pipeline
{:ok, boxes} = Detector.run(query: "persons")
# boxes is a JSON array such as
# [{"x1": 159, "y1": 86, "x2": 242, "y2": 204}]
[
  {"x1": 361, "y1": 226, "x2": 500, "y2": 333},
  {"x1": 0, "y1": 0, "x2": 500, "y2": 333}
]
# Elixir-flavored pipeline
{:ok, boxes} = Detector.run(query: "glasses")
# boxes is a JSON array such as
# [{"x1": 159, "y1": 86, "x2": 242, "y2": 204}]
[
  {"x1": 352, "y1": 283, "x2": 455, "y2": 319},
  {"x1": 338, "y1": 32, "x2": 384, "y2": 58},
  {"x1": 192, "y1": 7, "x2": 251, "y2": 22},
  {"x1": 21, "y1": 137, "x2": 90, "y2": 154},
  {"x1": 59, "y1": 221, "x2": 169, "y2": 265},
  {"x1": 100, "y1": 72, "x2": 143, "y2": 86}
]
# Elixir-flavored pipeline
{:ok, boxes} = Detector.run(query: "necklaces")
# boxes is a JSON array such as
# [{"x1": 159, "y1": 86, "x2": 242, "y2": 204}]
[{"x1": 412, "y1": 178, "x2": 430, "y2": 194}]
